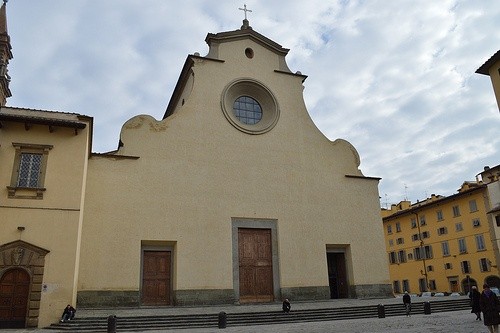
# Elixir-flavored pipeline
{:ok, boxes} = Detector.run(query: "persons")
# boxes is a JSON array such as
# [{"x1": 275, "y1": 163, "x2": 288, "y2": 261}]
[
  {"x1": 61, "y1": 304, "x2": 76, "y2": 322},
  {"x1": 469, "y1": 286, "x2": 482, "y2": 320},
  {"x1": 403, "y1": 291, "x2": 412, "y2": 317},
  {"x1": 282, "y1": 299, "x2": 291, "y2": 313},
  {"x1": 478, "y1": 283, "x2": 500, "y2": 333}
]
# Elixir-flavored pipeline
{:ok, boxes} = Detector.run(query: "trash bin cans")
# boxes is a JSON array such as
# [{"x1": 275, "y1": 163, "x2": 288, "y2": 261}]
[
  {"x1": 107, "y1": 314, "x2": 116, "y2": 333},
  {"x1": 377, "y1": 303, "x2": 386, "y2": 318},
  {"x1": 423, "y1": 300, "x2": 431, "y2": 315},
  {"x1": 218, "y1": 312, "x2": 226, "y2": 328}
]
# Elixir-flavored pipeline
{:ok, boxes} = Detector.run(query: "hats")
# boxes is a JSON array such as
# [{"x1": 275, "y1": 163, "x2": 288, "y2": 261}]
[{"x1": 404, "y1": 291, "x2": 408, "y2": 293}]
